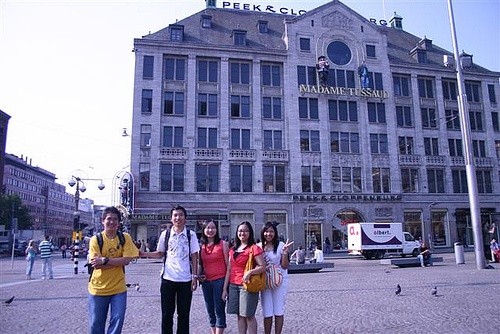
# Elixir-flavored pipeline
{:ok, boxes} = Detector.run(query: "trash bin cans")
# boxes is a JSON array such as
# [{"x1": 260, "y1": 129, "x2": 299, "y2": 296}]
[{"x1": 453, "y1": 242, "x2": 465, "y2": 264}]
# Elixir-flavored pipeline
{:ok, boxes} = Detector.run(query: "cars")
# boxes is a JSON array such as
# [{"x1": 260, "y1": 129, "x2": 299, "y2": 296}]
[{"x1": 9, "y1": 239, "x2": 28, "y2": 256}]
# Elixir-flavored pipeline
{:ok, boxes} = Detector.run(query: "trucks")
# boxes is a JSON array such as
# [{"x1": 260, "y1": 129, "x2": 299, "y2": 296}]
[{"x1": 346, "y1": 221, "x2": 423, "y2": 260}]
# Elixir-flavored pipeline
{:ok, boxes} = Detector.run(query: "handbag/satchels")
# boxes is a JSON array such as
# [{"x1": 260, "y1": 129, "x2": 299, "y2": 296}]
[
  {"x1": 242, "y1": 246, "x2": 266, "y2": 292},
  {"x1": 264, "y1": 253, "x2": 283, "y2": 289}
]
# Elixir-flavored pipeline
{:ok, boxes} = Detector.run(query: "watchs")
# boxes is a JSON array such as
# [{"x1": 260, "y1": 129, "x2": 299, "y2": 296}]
[
  {"x1": 192, "y1": 274, "x2": 197, "y2": 278},
  {"x1": 102, "y1": 257, "x2": 109, "y2": 265}
]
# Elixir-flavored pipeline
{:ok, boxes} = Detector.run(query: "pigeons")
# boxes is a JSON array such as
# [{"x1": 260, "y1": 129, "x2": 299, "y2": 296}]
[
  {"x1": 126, "y1": 283, "x2": 140, "y2": 291},
  {"x1": 395, "y1": 284, "x2": 401, "y2": 296},
  {"x1": 432, "y1": 287, "x2": 437, "y2": 296}
]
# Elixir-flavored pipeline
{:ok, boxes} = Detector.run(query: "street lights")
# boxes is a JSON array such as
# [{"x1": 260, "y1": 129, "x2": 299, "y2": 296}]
[
  {"x1": 67, "y1": 174, "x2": 106, "y2": 262},
  {"x1": 442, "y1": 49, "x2": 490, "y2": 270}
]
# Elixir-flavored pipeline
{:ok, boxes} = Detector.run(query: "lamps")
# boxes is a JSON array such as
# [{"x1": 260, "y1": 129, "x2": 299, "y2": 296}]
[{"x1": 122, "y1": 127, "x2": 127, "y2": 137}]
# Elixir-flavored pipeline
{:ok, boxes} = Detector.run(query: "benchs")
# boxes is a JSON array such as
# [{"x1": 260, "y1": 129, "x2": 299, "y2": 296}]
[
  {"x1": 390, "y1": 257, "x2": 443, "y2": 266},
  {"x1": 286, "y1": 262, "x2": 334, "y2": 274}
]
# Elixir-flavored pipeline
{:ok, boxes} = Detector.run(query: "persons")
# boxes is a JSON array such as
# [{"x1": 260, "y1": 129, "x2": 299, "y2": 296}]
[
  {"x1": 25, "y1": 240, "x2": 38, "y2": 275},
  {"x1": 88, "y1": 206, "x2": 139, "y2": 334},
  {"x1": 119, "y1": 178, "x2": 128, "y2": 205},
  {"x1": 61, "y1": 242, "x2": 67, "y2": 258},
  {"x1": 416, "y1": 227, "x2": 422, "y2": 241},
  {"x1": 482, "y1": 221, "x2": 498, "y2": 262},
  {"x1": 132, "y1": 239, "x2": 152, "y2": 251},
  {"x1": 358, "y1": 61, "x2": 370, "y2": 89},
  {"x1": 316, "y1": 56, "x2": 329, "y2": 87},
  {"x1": 417, "y1": 242, "x2": 433, "y2": 268},
  {"x1": 255, "y1": 222, "x2": 294, "y2": 334},
  {"x1": 39, "y1": 235, "x2": 54, "y2": 279},
  {"x1": 139, "y1": 205, "x2": 200, "y2": 334},
  {"x1": 198, "y1": 236, "x2": 261, "y2": 249},
  {"x1": 198, "y1": 218, "x2": 230, "y2": 334},
  {"x1": 281, "y1": 233, "x2": 331, "y2": 264},
  {"x1": 222, "y1": 221, "x2": 266, "y2": 334}
]
